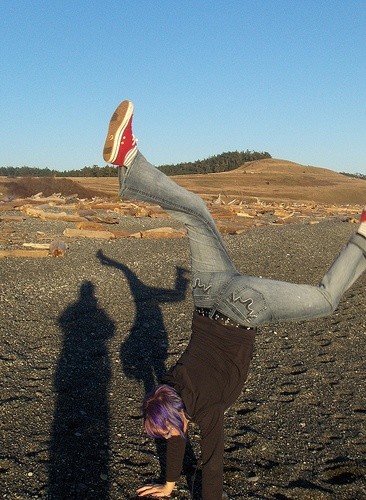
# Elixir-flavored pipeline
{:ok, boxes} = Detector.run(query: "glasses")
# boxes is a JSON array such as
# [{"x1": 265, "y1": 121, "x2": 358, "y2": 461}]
[{"x1": 161, "y1": 425, "x2": 173, "y2": 439}]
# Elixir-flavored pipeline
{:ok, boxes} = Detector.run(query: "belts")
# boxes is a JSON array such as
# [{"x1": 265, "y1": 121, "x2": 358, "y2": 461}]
[{"x1": 195, "y1": 306, "x2": 249, "y2": 330}]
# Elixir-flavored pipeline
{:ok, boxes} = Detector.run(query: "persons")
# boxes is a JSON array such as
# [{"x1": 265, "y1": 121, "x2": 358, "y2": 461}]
[{"x1": 102, "y1": 99, "x2": 365, "y2": 500}]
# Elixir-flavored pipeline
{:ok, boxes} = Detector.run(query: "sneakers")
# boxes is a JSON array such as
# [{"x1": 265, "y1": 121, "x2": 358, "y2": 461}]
[{"x1": 103, "y1": 100, "x2": 138, "y2": 166}]
